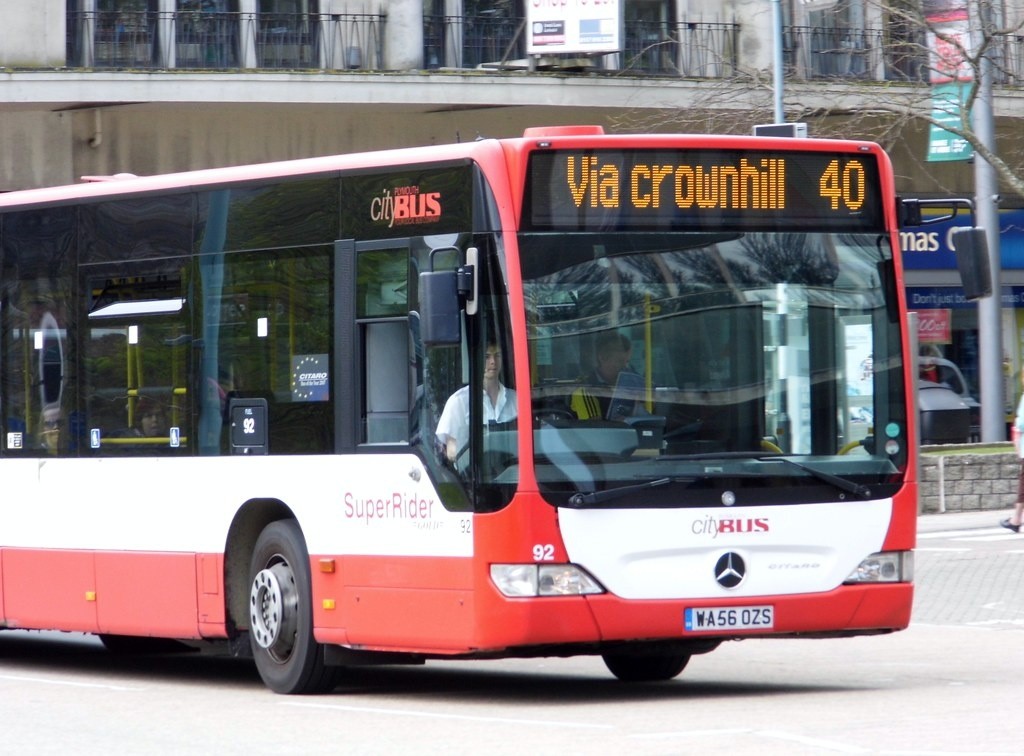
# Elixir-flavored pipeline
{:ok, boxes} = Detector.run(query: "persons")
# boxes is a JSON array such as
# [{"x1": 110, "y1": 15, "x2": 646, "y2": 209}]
[
  {"x1": 435, "y1": 341, "x2": 517, "y2": 472},
  {"x1": 1000, "y1": 394, "x2": 1024, "y2": 533},
  {"x1": 93, "y1": 399, "x2": 187, "y2": 457},
  {"x1": 571, "y1": 333, "x2": 632, "y2": 420},
  {"x1": 31, "y1": 402, "x2": 70, "y2": 458}
]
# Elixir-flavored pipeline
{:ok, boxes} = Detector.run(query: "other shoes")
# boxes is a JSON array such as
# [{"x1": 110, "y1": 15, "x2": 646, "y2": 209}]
[{"x1": 1000, "y1": 517, "x2": 1020, "y2": 533}]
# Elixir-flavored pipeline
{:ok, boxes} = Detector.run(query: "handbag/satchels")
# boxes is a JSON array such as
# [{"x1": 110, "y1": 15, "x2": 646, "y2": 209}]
[{"x1": 1010, "y1": 414, "x2": 1023, "y2": 443}]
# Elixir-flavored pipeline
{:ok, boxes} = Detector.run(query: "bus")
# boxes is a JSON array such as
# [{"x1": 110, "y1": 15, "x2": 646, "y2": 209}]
[{"x1": 2, "y1": 124, "x2": 993, "y2": 696}]
